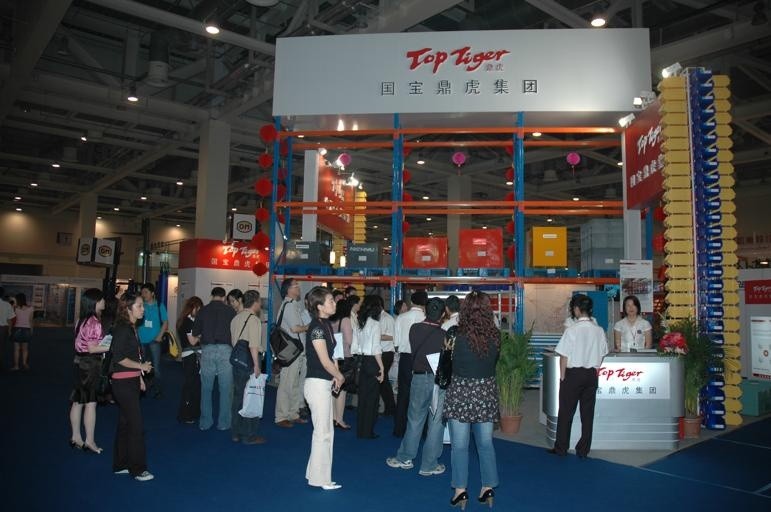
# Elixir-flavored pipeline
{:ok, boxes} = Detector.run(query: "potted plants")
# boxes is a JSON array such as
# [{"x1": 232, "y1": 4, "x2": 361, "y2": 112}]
[
  {"x1": 493, "y1": 318, "x2": 543, "y2": 435},
  {"x1": 663, "y1": 315, "x2": 718, "y2": 437}
]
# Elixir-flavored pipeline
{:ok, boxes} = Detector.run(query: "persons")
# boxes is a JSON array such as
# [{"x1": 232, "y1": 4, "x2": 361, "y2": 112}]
[
  {"x1": 443, "y1": 289, "x2": 503, "y2": 507},
  {"x1": 275, "y1": 278, "x2": 460, "y2": 447},
  {"x1": 502, "y1": 316, "x2": 508, "y2": 329},
  {"x1": 137, "y1": 282, "x2": 167, "y2": 399},
  {"x1": 614, "y1": 295, "x2": 653, "y2": 352},
  {"x1": 563, "y1": 295, "x2": 598, "y2": 333},
  {"x1": 303, "y1": 285, "x2": 347, "y2": 491},
  {"x1": 107, "y1": 288, "x2": 157, "y2": 483},
  {"x1": 1, "y1": 290, "x2": 33, "y2": 370},
  {"x1": 385, "y1": 297, "x2": 446, "y2": 476},
  {"x1": 545, "y1": 295, "x2": 611, "y2": 460},
  {"x1": 70, "y1": 287, "x2": 113, "y2": 456},
  {"x1": 176, "y1": 287, "x2": 268, "y2": 444}
]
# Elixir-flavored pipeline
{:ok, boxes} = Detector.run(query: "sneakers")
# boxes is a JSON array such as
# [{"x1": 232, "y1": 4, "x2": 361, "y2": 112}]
[
  {"x1": 135, "y1": 470, "x2": 154, "y2": 482},
  {"x1": 115, "y1": 468, "x2": 129, "y2": 474},
  {"x1": 386, "y1": 457, "x2": 414, "y2": 469},
  {"x1": 419, "y1": 463, "x2": 445, "y2": 476}
]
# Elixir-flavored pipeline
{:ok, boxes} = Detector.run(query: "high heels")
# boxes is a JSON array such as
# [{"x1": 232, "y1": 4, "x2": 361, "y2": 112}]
[
  {"x1": 82, "y1": 441, "x2": 105, "y2": 455},
  {"x1": 478, "y1": 489, "x2": 495, "y2": 509},
  {"x1": 308, "y1": 480, "x2": 343, "y2": 490},
  {"x1": 305, "y1": 475, "x2": 337, "y2": 485},
  {"x1": 70, "y1": 438, "x2": 86, "y2": 450},
  {"x1": 450, "y1": 492, "x2": 469, "y2": 510},
  {"x1": 335, "y1": 419, "x2": 352, "y2": 430}
]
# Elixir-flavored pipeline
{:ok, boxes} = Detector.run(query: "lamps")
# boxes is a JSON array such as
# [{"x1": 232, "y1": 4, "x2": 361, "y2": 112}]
[
  {"x1": 662, "y1": 62, "x2": 682, "y2": 78},
  {"x1": 632, "y1": 89, "x2": 656, "y2": 111},
  {"x1": 205, "y1": 8, "x2": 223, "y2": 35},
  {"x1": 316, "y1": 142, "x2": 364, "y2": 190},
  {"x1": 127, "y1": 79, "x2": 139, "y2": 103},
  {"x1": 80, "y1": 129, "x2": 89, "y2": 143},
  {"x1": 618, "y1": 112, "x2": 635, "y2": 128},
  {"x1": 176, "y1": 176, "x2": 184, "y2": 185}
]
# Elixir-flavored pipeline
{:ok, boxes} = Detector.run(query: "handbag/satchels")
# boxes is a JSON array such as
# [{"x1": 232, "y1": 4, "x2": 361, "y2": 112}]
[
  {"x1": 339, "y1": 355, "x2": 361, "y2": 393},
  {"x1": 434, "y1": 348, "x2": 458, "y2": 388},
  {"x1": 69, "y1": 349, "x2": 104, "y2": 377},
  {"x1": 238, "y1": 374, "x2": 269, "y2": 419},
  {"x1": 161, "y1": 331, "x2": 179, "y2": 359},
  {"x1": 332, "y1": 332, "x2": 344, "y2": 360},
  {"x1": 229, "y1": 339, "x2": 263, "y2": 371}
]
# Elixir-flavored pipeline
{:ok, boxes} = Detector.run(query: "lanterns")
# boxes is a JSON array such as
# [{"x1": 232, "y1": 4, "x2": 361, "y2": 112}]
[
  {"x1": 505, "y1": 191, "x2": 515, "y2": 220},
  {"x1": 403, "y1": 192, "x2": 413, "y2": 211},
  {"x1": 403, "y1": 221, "x2": 410, "y2": 233},
  {"x1": 642, "y1": 210, "x2": 646, "y2": 220},
  {"x1": 507, "y1": 244, "x2": 515, "y2": 268},
  {"x1": 253, "y1": 124, "x2": 290, "y2": 281},
  {"x1": 506, "y1": 139, "x2": 515, "y2": 157},
  {"x1": 404, "y1": 140, "x2": 412, "y2": 158},
  {"x1": 403, "y1": 170, "x2": 412, "y2": 184},
  {"x1": 506, "y1": 222, "x2": 515, "y2": 238},
  {"x1": 453, "y1": 152, "x2": 465, "y2": 167},
  {"x1": 506, "y1": 168, "x2": 514, "y2": 183},
  {"x1": 653, "y1": 234, "x2": 668, "y2": 253},
  {"x1": 658, "y1": 265, "x2": 670, "y2": 279},
  {"x1": 654, "y1": 207, "x2": 666, "y2": 222},
  {"x1": 339, "y1": 152, "x2": 352, "y2": 167},
  {"x1": 568, "y1": 152, "x2": 580, "y2": 166}
]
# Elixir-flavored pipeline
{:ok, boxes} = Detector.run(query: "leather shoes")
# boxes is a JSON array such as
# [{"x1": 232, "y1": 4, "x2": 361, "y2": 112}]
[
  {"x1": 243, "y1": 435, "x2": 266, "y2": 446},
  {"x1": 232, "y1": 433, "x2": 252, "y2": 443},
  {"x1": 547, "y1": 447, "x2": 567, "y2": 457},
  {"x1": 274, "y1": 406, "x2": 312, "y2": 428},
  {"x1": 357, "y1": 432, "x2": 380, "y2": 439},
  {"x1": 576, "y1": 450, "x2": 586, "y2": 458}
]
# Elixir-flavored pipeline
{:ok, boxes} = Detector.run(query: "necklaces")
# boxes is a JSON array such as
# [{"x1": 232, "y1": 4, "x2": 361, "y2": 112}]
[{"x1": 629, "y1": 323, "x2": 637, "y2": 346}]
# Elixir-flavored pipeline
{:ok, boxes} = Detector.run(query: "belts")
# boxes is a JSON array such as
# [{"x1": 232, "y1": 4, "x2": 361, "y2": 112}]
[{"x1": 413, "y1": 370, "x2": 432, "y2": 375}]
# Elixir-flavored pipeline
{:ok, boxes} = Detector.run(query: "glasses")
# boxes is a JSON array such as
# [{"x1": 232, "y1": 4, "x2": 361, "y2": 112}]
[{"x1": 288, "y1": 284, "x2": 300, "y2": 288}]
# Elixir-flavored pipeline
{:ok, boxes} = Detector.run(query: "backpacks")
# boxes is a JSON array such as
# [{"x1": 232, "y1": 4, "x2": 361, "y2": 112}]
[{"x1": 269, "y1": 301, "x2": 304, "y2": 367}]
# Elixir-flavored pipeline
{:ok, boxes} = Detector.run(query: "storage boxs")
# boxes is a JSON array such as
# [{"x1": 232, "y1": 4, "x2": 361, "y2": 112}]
[
  {"x1": 347, "y1": 243, "x2": 383, "y2": 269},
  {"x1": 286, "y1": 239, "x2": 329, "y2": 266},
  {"x1": 526, "y1": 226, "x2": 568, "y2": 269},
  {"x1": 402, "y1": 237, "x2": 448, "y2": 268},
  {"x1": 459, "y1": 227, "x2": 504, "y2": 269},
  {"x1": 580, "y1": 218, "x2": 624, "y2": 272}
]
